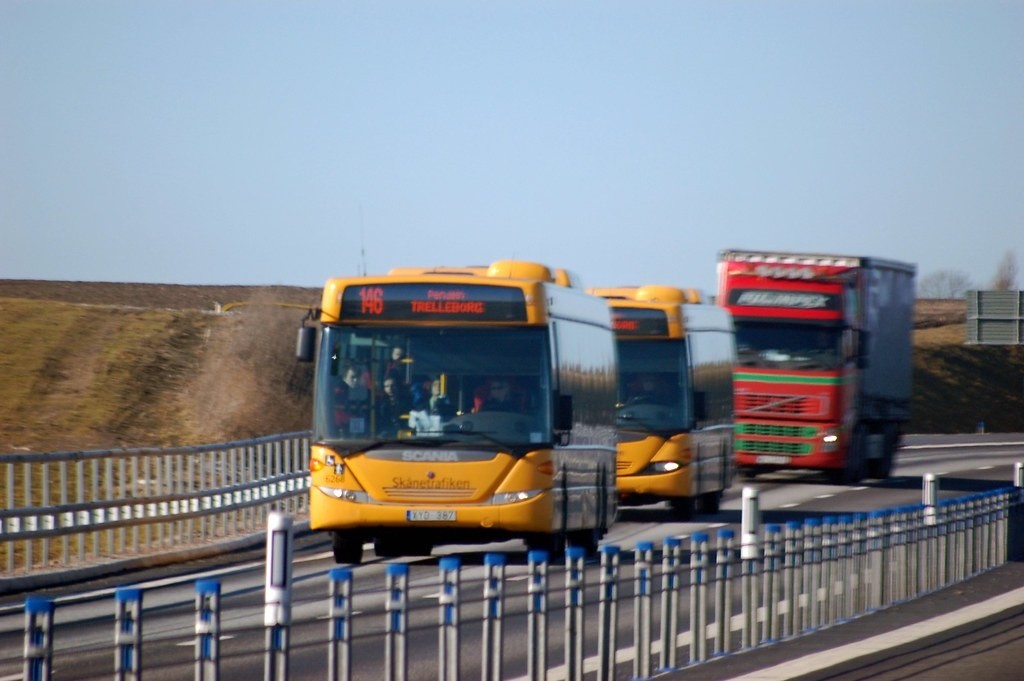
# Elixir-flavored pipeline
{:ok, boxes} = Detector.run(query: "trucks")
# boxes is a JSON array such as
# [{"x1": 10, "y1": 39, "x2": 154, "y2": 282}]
[{"x1": 715, "y1": 249, "x2": 918, "y2": 486}]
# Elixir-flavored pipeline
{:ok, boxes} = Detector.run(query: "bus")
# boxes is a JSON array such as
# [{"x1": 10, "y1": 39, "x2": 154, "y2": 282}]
[
  {"x1": 583, "y1": 285, "x2": 735, "y2": 525},
  {"x1": 295, "y1": 260, "x2": 623, "y2": 566}
]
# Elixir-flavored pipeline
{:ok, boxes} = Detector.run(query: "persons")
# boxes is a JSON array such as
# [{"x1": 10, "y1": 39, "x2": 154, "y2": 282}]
[
  {"x1": 332, "y1": 342, "x2": 484, "y2": 441},
  {"x1": 480, "y1": 375, "x2": 531, "y2": 416},
  {"x1": 637, "y1": 375, "x2": 666, "y2": 404}
]
